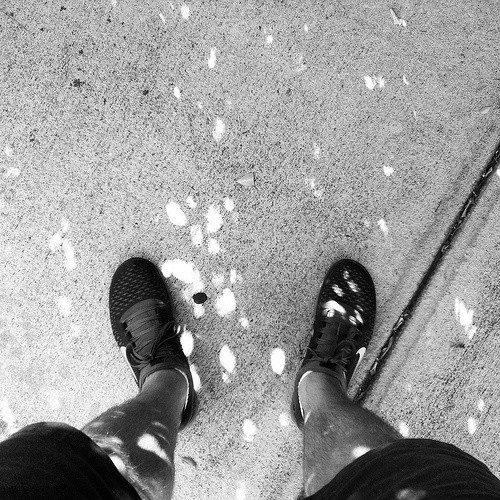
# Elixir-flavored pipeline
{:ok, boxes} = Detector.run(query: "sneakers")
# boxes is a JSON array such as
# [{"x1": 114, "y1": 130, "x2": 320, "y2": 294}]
[
  {"x1": 109, "y1": 257, "x2": 198, "y2": 431},
  {"x1": 290, "y1": 259, "x2": 376, "y2": 435}
]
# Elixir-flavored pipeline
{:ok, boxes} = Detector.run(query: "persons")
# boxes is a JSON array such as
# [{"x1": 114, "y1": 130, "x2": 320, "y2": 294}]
[{"x1": 1, "y1": 257, "x2": 500, "y2": 500}]
